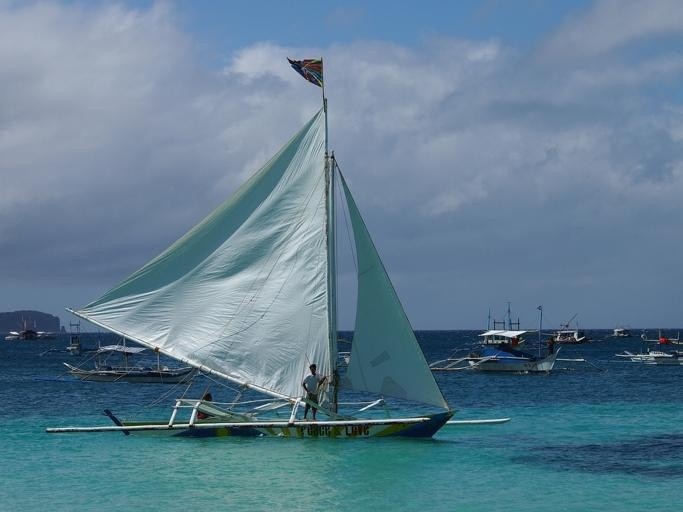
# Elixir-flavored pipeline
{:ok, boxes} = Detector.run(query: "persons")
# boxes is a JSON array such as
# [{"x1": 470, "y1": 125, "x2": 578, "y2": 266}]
[
  {"x1": 303, "y1": 364, "x2": 326, "y2": 422},
  {"x1": 548, "y1": 335, "x2": 553, "y2": 354},
  {"x1": 198, "y1": 394, "x2": 214, "y2": 420}
]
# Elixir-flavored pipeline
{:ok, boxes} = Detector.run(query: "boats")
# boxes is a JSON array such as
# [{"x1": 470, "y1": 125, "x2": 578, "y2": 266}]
[
  {"x1": 615, "y1": 329, "x2": 683, "y2": 366},
  {"x1": 66, "y1": 320, "x2": 83, "y2": 357},
  {"x1": 554, "y1": 313, "x2": 593, "y2": 344},
  {"x1": 428, "y1": 300, "x2": 562, "y2": 375},
  {"x1": 4, "y1": 329, "x2": 65, "y2": 341},
  {"x1": 611, "y1": 327, "x2": 632, "y2": 338},
  {"x1": 62, "y1": 330, "x2": 195, "y2": 384}
]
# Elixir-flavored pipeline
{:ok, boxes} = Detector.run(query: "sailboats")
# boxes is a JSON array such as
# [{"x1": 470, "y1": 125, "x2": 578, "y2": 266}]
[{"x1": 45, "y1": 54, "x2": 512, "y2": 439}]
